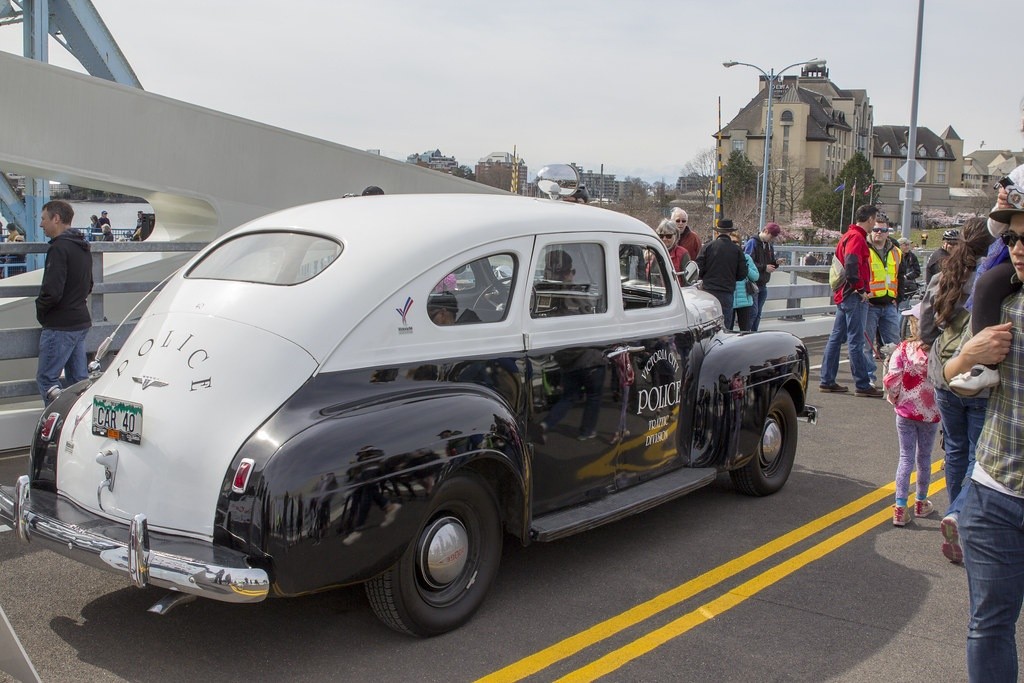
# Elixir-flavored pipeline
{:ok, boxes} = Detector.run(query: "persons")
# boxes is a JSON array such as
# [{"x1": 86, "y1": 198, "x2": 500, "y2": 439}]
[
  {"x1": 344, "y1": 186, "x2": 384, "y2": 198},
  {"x1": 642, "y1": 218, "x2": 691, "y2": 287},
  {"x1": 819, "y1": 164, "x2": 1024, "y2": 683},
  {"x1": 667, "y1": 206, "x2": 701, "y2": 261},
  {"x1": 1, "y1": 211, "x2": 155, "y2": 277},
  {"x1": 33, "y1": 200, "x2": 95, "y2": 410},
  {"x1": 214, "y1": 246, "x2": 807, "y2": 568},
  {"x1": 696, "y1": 219, "x2": 786, "y2": 334}
]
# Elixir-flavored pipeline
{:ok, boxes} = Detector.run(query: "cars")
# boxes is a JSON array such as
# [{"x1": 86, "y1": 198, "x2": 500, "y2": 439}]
[{"x1": 14, "y1": 193, "x2": 820, "y2": 643}]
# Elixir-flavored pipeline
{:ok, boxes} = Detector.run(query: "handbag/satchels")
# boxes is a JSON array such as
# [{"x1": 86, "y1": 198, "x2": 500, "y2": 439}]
[{"x1": 745, "y1": 280, "x2": 759, "y2": 295}]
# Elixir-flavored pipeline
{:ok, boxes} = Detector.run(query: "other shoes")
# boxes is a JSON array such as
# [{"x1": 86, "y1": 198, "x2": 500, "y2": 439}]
[
  {"x1": 854, "y1": 388, "x2": 882, "y2": 397},
  {"x1": 948, "y1": 365, "x2": 999, "y2": 394},
  {"x1": 608, "y1": 429, "x2": 630, "y2": 444},
  {"x1": 819, "y1": 383, "x2": 848, "y2": 392}
]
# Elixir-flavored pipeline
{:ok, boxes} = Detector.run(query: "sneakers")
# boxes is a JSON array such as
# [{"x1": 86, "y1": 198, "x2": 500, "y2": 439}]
[
  {"x1": 893, "y1": 507, "x2": 911, "y2": 525},
  {"x1": 914, "y1": 500, "x2": 934, "y2": 517},
  {"x1": 536, "y1": 422, "x2": 547, "y2": 444},
  {"x1": 940, "y1": 513, "x2": 964, "y2": 563},
  {"x1": 343, "y1": 532, "x2": 362, "y2": 545},
  {"x1": 578, "y1": 431, "x2": 597, "y2": 440},
  {"x1": 380, "y1": 504, "x2": 401, "y2": 526}
]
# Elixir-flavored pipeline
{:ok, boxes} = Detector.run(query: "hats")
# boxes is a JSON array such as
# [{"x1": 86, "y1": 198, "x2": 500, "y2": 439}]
[
  {"x1": 767, "y1": 222, "x2": 781, "y2": 236},
  {"x1": 989, "y1": 209, "x2": 1024, "y2": 224},
  {"x1": 713, "y1": 220, "x2": 738, "y2": 232},
  {"x1": 901, "y1": 303, "x2": 920, "y2": 319},
  {"x1": 427, "y1": 291, "x2": 458, "y2": 313},
  {"x1": 897, "y1": 239, "x2": 913, "y2": 246},
  {"x1": 102, "y1": 211, "x2": 107, "y2": 214}
]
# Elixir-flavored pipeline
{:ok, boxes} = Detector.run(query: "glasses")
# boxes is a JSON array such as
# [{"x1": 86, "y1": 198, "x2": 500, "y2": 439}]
[
  {"x1": 658, "y1": 234, "x2": 676, "y2": 239},
  {"x1": 675, "y1": 220, "x2": 687, "y2": 223},
  {"x1": 569, "y1": 268, "x2": 576, "y2": 276},
  {"x1": 872, "y1": 228, "x2": 888, "y2": 233},
  {"x1": 731, "y1": 240, "x2": 739, "y2": 244},
  {"x1": 1000, "y1": 230, "x2": 1024, "y2": 247}
]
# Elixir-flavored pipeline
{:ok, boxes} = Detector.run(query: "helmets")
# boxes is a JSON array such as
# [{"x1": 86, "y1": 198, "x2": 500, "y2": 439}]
[
  {"x1": 942, "y1": 230, "x2": 959, "y2": 241},
  {"x1": 875, "y1": 213, "x2": 890, "y2": 223}
]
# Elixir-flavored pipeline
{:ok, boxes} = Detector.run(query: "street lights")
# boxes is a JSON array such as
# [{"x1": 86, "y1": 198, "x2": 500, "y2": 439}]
[
  {"x1": 755, "y1": 168, "x2": 786, "y2": 223},
  {"x1": 721, "y1": 57, "x2": 828, "y2": 233}
]
